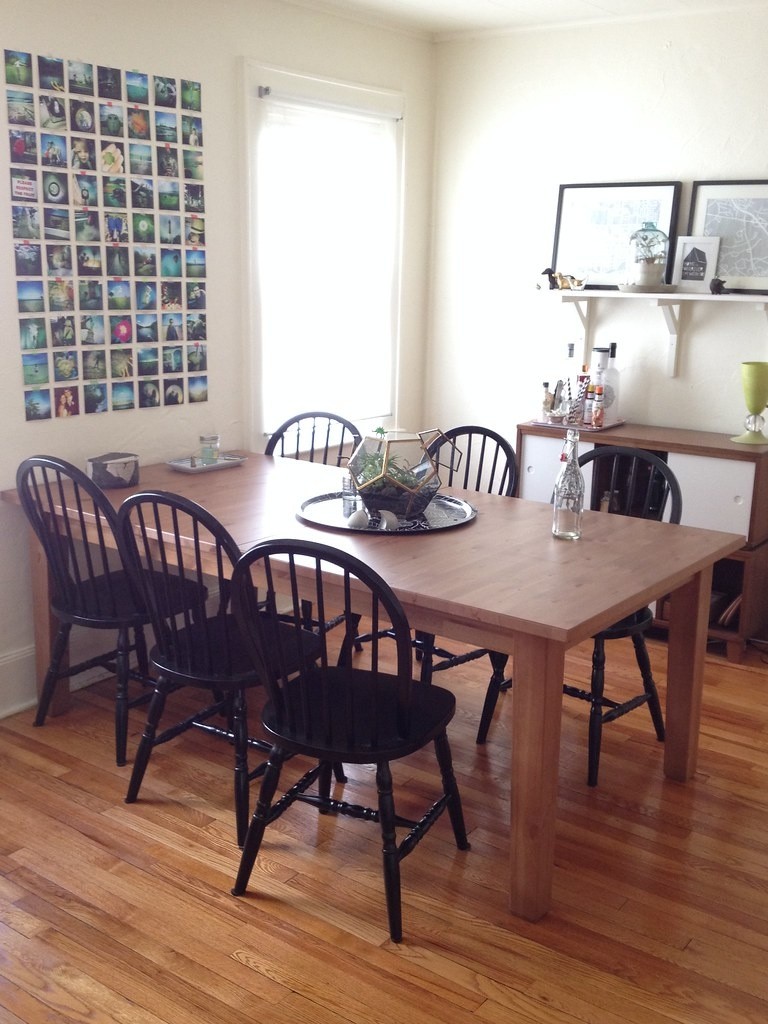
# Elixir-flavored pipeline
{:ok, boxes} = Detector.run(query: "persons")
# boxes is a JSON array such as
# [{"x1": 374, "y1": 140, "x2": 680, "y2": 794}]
[
  {"x1": 70, "y1": 138, "x2": 98, "y2": 172},
  {"x1": 166, "y1": 318, "x2": 178, "y2": 340},
  {"x1": 186, "y1": 285, "x2": 205, "y2": 308},
  {"x1": 188, "y1": 128, "x2": 200, "y2": 145},
  {"x1": 161, "y1": 284, "x2": 170, "y2": 311},
  {"x1": 11, "y1": 58, "x2": 23, "y2": 80},
  {"x1": 57, "y1": 388, "x2": 77, "y2": 418}
]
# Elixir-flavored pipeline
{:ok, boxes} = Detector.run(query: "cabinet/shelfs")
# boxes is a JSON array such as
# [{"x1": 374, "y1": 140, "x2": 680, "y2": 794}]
[{"x1": 516, "y1": 423, "x2": 768, "y2": 663}]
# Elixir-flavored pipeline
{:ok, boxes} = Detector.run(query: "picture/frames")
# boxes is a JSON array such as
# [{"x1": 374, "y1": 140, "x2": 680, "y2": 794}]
[
  {"x1": 550, "y1": 181, "x2": 682, "y2": 290},
  {"x1": 687, "y1": 179, "x2": 768, "y2": 295}
]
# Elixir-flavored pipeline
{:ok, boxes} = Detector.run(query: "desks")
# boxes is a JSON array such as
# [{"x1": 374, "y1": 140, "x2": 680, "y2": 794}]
[
  {"x1": 0, "y1": 450, "x2": 746, "y2": 920},
  {"x1": 562, "y1": 291, "x2": 768, "y2": 378}
]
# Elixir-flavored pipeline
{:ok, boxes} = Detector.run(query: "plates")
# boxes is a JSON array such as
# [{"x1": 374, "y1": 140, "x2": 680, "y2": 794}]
[
  {"x1": 616, "y1": 284, "x2": 677, "y2": 292},
  {"x1": 166, "y1": 454, "x2": 248, "y2": 474}
]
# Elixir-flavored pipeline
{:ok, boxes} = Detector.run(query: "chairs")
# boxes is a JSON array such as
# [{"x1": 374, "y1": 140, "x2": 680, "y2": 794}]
[{"x1": 16, "y1": 411, "x2": 682, "y2": 943}]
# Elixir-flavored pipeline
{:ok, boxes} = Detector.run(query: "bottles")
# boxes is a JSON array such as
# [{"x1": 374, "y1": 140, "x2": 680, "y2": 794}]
[
  {"x1": 200, "y1": 434, "x2": 219, "y2": 466},
  {"x1": 552, "y1": 430, "x2": 585, "y2": 540},
  {"x1": 541, "y1": 365, "x2": 606, "y2": 429}
]
